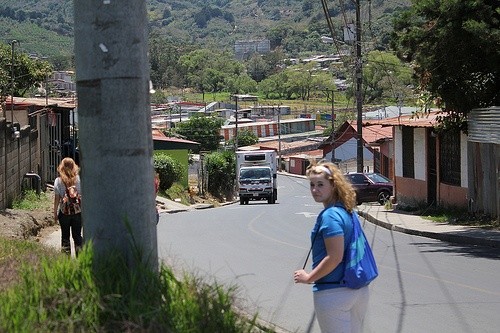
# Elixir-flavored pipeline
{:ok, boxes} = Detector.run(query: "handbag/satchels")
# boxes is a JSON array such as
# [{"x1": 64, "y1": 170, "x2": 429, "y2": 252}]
[
  {"x1": 60, "y1": 177, "x2": 81, "y2": 216},
  {"x1": 300, "y1": 206, "x2": 378, "y2": 290}
]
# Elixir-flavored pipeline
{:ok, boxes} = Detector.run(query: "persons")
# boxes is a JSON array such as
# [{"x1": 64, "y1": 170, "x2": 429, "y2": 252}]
[
  {"x1": 154, "y1": 170, "x2": 159, "y2": 226},
  {"x1": 295, "y1": 157, "x2": 375, "y2": 333},
  {"x1": 51, "y1": 158, "x2": 83, "y2": 259}
]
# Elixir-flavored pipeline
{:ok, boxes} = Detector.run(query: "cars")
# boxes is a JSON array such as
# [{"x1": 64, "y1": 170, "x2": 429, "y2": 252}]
[{"x1": 343, "y1": 172, "x2": 393, "y2": 206}]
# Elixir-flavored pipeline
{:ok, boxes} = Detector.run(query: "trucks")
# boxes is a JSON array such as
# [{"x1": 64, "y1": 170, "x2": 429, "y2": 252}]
[{"x1": 234, "y1": 149, "x2": 279, "y2": 205}]
